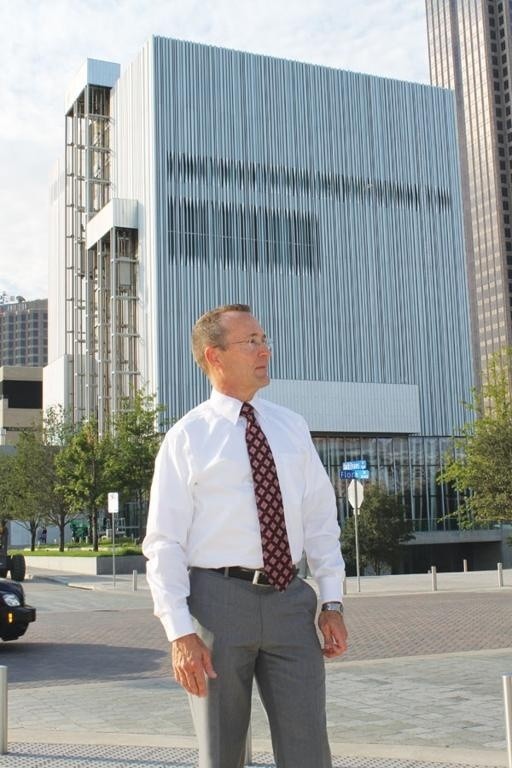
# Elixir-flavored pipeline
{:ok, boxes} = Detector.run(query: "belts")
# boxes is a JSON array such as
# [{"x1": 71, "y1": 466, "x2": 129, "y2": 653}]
[{"x1": 213, "y1": 567, "x2": 300, "y2": 587}]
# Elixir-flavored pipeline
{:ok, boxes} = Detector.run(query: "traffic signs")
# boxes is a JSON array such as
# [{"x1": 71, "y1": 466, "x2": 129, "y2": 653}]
[
  {"x1": 353, "y1": 469, "x2": 370, "y2": 479},
  {"x1": 341, "y1": 459, "x2": 368, "y2": 471},
  {"x1": 340, "y1": 471, "x2": 359, "y2": 480}
]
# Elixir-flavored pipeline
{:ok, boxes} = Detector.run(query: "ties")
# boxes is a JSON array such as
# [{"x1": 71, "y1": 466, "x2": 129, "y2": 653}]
[{"x1": 240, "y1": 402, "x2": 294, "y2": 594}]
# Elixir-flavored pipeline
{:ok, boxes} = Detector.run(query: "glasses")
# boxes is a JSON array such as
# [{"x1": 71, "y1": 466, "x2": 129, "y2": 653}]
[{"x1": 212, "y1": 336, "x2": 274, "y2": 349}]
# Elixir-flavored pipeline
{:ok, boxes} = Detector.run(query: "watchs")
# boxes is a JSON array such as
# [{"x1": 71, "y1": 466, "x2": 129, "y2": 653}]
[{"x1": 322, "y1": 602, "x2": 344, "y2": 615}]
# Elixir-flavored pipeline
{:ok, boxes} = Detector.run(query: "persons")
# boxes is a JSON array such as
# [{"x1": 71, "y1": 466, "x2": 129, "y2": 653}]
[{"x1": 142, "y1": 304, "x2": 347, "y2": 768}]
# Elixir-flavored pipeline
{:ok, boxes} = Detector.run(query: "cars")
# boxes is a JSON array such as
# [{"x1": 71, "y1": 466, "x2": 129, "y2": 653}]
[{"x1": 0, "y1": 576, "x2": 37, "y2": 643}]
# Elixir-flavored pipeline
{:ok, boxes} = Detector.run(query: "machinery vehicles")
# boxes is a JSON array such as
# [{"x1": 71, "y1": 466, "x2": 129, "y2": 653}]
[{"x1": 0, "y1": 519, "x2": 27, "y2": 581}]
